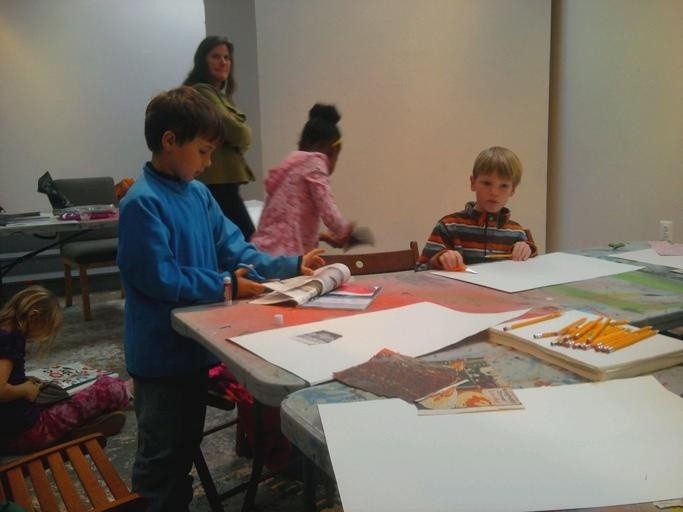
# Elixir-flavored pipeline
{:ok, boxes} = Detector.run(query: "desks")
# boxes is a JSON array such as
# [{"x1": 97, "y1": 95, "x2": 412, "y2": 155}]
[
  {"x1": 280, "y1": 340, "x2": 683, "y2": 512},
  {"x1": 565, "y1": 240, "x2": 683, "y2": 280},
  {"x1": 169, "y1": 251, "x2": 683, "y2": 511},
  {"x1": 0, "y1": 205, "x2": 122, "y2": 286}
]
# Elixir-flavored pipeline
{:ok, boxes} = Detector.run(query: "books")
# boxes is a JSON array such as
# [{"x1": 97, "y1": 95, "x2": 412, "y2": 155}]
[
  {"x1": 414, "y1": 350, "x2": 524, "y2": 415},
  {"x1": 489, "y1": 310, "x2": 683, "y2": 384},
  {"x1": 248, "y1": 262, "x2": 382, "y2": 311}
]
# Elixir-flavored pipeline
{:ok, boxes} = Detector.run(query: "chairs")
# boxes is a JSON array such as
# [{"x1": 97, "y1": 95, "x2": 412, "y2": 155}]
[
  {"x1": 50, "y1": 176, "x2": 125, "y2": 321},
  {"x1": 309, "y1": 240, "x2": 420, "y2": 276},
  {"x1": 0, "y1": 432, "x2": 144, "y2": 512}
]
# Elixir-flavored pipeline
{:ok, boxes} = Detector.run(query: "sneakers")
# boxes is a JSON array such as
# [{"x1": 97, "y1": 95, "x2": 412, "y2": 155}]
[{"x1": 63, "y1": 408, "x2": 128, "y2": 445}]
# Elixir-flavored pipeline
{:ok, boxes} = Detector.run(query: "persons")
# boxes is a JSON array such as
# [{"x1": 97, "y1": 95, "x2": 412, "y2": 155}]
[
  {"x1": 182, "y1": 37, "x2": 255, "y2": 243},
  {"x1": 237, "y1": 104, "x2": 355, "y2": 468},
  {"x1": 0, "y1": 285, "x2": 135, "y2": 455},
  {"x1": 117, "y1": 87, "x2": 326, "y2": 512},
  {"x1": 415, "y1": 148, "x2": 537, "y2": 271}
]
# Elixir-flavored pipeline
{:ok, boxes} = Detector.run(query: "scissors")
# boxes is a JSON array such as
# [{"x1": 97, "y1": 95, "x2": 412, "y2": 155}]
[
  {"x1": 235, "y1": 263, "x2": 280, "y2": 284},
  {"x1": 454, "y1": 262, "x2": 478, "y2": 274},
  {"x1": 32, "y1": 381, "x2": 50, "y2": 391}
]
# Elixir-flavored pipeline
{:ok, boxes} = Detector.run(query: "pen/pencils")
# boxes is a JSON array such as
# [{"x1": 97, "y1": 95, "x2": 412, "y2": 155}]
[
  {"x1": 533, "y1": 314, "x2": 661, "y2": 352},
  {"x1": 484, "y1": 254, "x2": 513, "y2": 259},
  {"x1": 503, "y1": 312, "x2": 563, "y2": 332}
]
